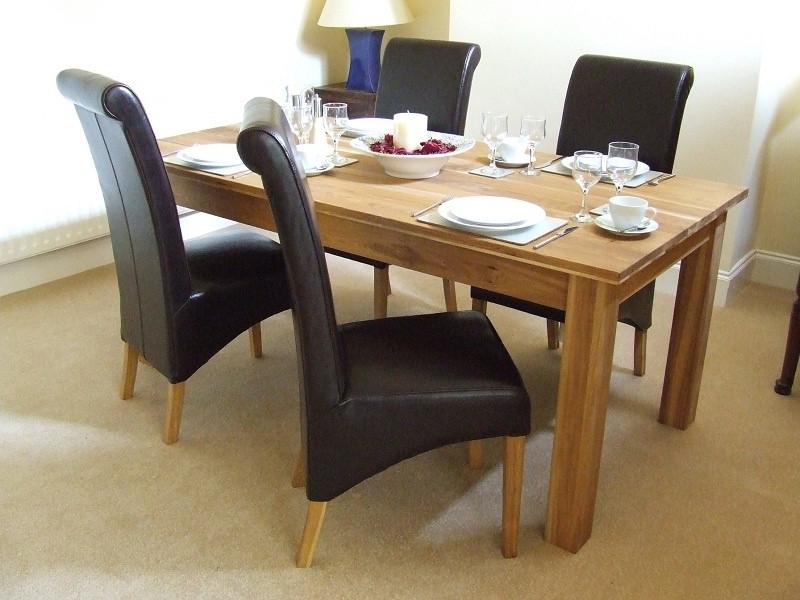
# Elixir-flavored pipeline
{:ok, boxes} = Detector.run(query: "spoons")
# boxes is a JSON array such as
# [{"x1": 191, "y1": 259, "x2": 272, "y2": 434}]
[
  {"x1": 617, "y1": 222, "x2": 650, "y2": 232},
  {"x1": 319, "y1": 164, "x2": 329, "y2": 169}
]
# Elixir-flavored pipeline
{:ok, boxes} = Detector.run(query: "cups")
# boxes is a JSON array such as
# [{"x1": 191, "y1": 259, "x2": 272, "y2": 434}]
[
  {"x1": 500, "y1": 137, "x2": 527, "y2": 162},
  {"x1": 296, "y1": 145, "x2": 319, "y2": 169},
  {"x1": 609, "y1": 196, "x2": 656, "y2": 229}
]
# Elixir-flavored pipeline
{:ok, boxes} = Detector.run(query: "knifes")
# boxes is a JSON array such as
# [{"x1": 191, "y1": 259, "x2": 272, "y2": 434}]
[{"x1": 533, "y1": 227, "x2": 578, "y2": 249}]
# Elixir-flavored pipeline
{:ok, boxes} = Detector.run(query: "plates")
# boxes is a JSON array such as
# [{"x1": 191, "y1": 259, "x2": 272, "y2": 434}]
[
  {"x1": 347, "y1": 118, "x2": 394, "y2": 133},
  {"x1": 561, "y1": 155, "x2": 650, "y2": 178},
  {"x1": 438, "y1": 196, "x2": 546, "y2": 231},
  {"x1": 305, "y1": 163, "x2": 334, "y2": 176},
  {"x1": 488, "y1": 152, "x2": 536, "y2": 167},
  {"x1": 594, "y1": 214, "x2": 659, "y2": 236},
  {"x1": 177, "y1": 144, "x2": 243, "y2": 168}
]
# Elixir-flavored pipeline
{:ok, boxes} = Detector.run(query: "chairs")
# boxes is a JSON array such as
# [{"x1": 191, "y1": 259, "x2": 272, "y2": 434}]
[
  {"x1": 235, "y1": 97, "x2": 531, "y2": 568},
  {"x1": 372, "y1": 37, "x2": 482, "y2": 321},
  {"x1": 471, "y1": 54, "x2": 695, "y2": 378},
  {"x1": 55, "y1": 68, "x2": 292, "y2": 446}
]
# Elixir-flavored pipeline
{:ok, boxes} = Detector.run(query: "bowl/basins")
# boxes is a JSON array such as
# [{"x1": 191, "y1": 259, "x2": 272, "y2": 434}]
[{"x1": 350, "y1": 132, "x2": 476, "y2": 179}]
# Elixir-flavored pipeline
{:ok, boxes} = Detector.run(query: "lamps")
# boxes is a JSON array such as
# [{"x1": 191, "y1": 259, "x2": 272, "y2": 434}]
[{"x1": 316, "y1": 0, "x2": 415, "y2": 93}]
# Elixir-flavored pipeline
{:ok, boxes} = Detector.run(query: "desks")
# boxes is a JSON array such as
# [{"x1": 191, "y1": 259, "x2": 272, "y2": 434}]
[{"x1": 313, "y1": 81, "x2": 378, "y2": 120}]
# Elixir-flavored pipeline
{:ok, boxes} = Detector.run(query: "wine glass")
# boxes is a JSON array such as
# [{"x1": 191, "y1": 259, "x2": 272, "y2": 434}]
[
  {"x1": 519, "y1": 116, "x2": 546, "y2": 176},
  {"x1": 323, "y1": 103, "x2": 350, "y2": 165},
  {"x1": 606, "y1": 142, "x2": 639, "y2": 196},
  {"x1": 481, "y1": 113, "x2": 508, "y2": 175},
  {"x1": 569, "y1": 150, "x2": 602, "y2": 222},
  {"x1": 289, "y1": 94, "x2": 313, "y2": 144}
]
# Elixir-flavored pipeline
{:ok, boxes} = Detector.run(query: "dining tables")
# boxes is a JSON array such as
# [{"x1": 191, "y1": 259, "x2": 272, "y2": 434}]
[{"x1": 155, "y1": 116, "x2": 750, "y2": 555}]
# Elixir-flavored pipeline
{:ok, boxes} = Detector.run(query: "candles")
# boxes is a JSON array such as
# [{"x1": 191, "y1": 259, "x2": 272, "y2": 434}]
[{"x1": 392, "y1": 108, "x2": 428, "y2": 153}]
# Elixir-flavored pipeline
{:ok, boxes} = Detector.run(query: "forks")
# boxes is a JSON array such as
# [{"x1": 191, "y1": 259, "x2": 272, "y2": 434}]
[{"x1": 647, "y1": 174, "x2": 676, "y2": 185}]
